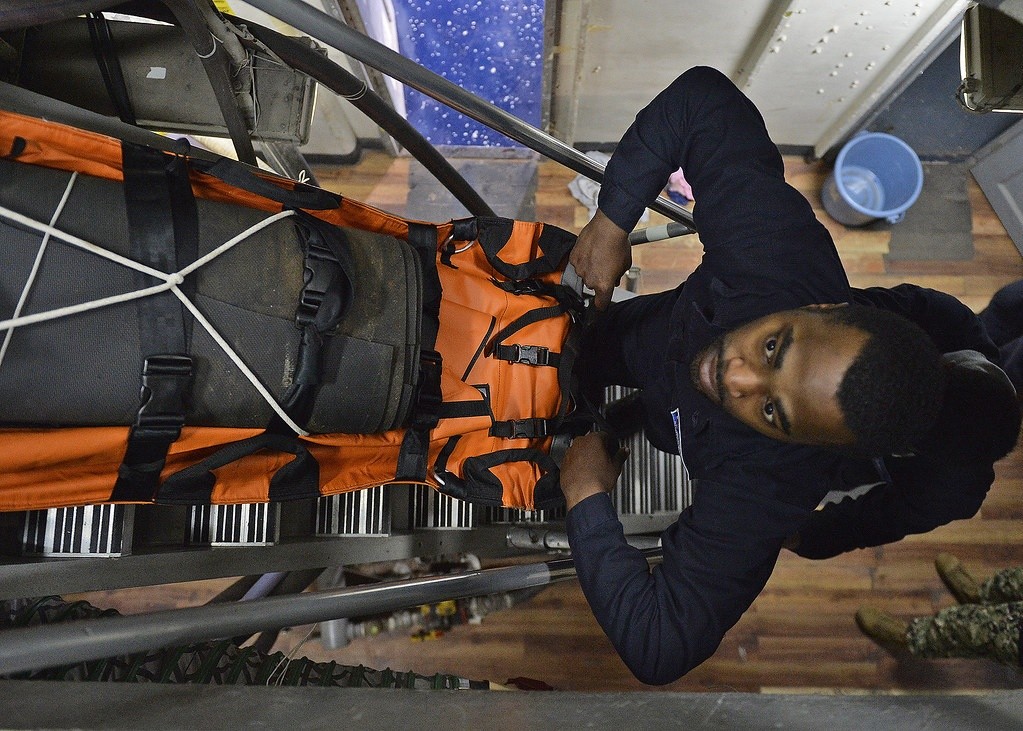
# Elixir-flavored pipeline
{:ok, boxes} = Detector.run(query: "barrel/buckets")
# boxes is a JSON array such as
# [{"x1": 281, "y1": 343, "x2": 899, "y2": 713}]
[{"x1": 822, "y1": 130, "x2": 924, "y2": 228}]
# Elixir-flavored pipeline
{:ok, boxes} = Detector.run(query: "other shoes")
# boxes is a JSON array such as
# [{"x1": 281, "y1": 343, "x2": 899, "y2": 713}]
[
  {"x1": 855, "y1": 606, "x2": 913, "y2": 649},
  {"x1": 935, "y1": 552, "x2": 981, "y2": 604}
]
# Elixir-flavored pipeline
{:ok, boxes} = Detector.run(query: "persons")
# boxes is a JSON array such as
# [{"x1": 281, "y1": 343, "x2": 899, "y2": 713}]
[
  {"x1": 781, "y1": 283, "x2": 1017, "y2": 565},
  {"x1": 977, "y1": 279, "x2": 1023, "y2": 416},
  {"x1": 560, "y1": 65, "x2": 944, "y2": 686}
]
